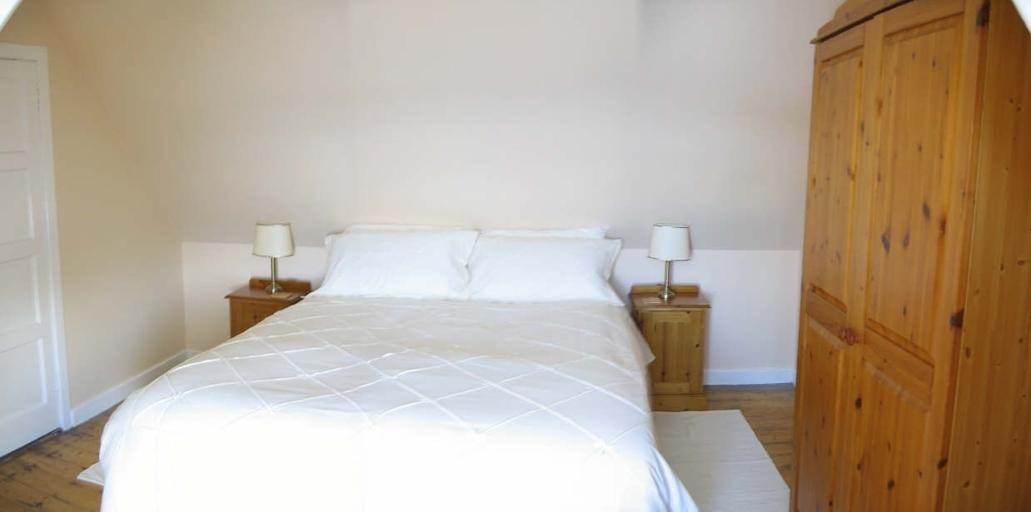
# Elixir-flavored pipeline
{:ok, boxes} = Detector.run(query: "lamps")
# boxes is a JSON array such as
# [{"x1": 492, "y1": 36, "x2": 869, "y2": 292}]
[
  {"x1": 648, "y1": 223, "x2": 692, "y2": 300},
  {"x1": 252, "y1": 220, "x2": 295, "y2": 292}
]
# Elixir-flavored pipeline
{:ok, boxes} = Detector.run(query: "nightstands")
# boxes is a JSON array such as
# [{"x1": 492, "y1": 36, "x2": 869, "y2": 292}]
[
  {"x1": 224, "y1": 277, "x2": 312, "y2": 338},
  {"x1": 628, "y1": 284, "x2": 712, "y2": 411}
]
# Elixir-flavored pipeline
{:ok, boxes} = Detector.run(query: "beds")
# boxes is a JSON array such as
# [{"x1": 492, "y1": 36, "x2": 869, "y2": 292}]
[{"x1": 96, "y1": 293, "x2": 700, "y2": 512}]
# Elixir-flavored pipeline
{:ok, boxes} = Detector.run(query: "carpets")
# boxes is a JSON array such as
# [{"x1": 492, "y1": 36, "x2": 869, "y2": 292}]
[{"x1": 653, "y1": 408, "x2": 791, "y2": 512}]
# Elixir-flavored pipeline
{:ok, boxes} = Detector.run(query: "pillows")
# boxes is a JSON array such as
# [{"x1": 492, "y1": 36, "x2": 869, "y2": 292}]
[
  {"x1": 456, "y1": 232, "x2": 629, "y2": 307},
  {"x1": 315, "y1": 229, "x2": 479, "y2": 301}
]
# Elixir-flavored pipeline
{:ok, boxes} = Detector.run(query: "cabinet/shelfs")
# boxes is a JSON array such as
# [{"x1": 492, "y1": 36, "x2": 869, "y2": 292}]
[{"x1": 788, "y1": 0, "x2": 1031, "y2": 511}]
